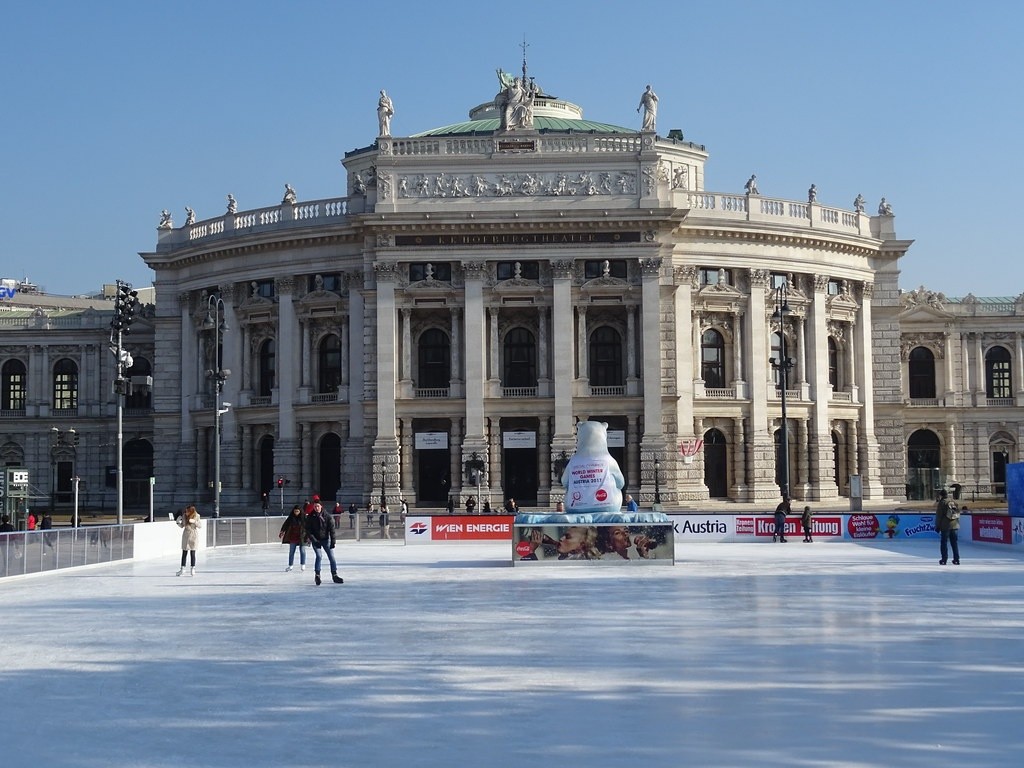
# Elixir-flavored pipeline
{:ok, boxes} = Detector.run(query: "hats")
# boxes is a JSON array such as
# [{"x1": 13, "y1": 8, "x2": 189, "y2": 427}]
[{"x1": 939, "y1": 489, "x2": 947, "y2": 496}]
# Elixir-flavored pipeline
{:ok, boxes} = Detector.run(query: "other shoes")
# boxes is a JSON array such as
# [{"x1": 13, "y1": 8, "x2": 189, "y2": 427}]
[
  {"x1": 780, "y1": 539, "x2": 787, "y2": 543},
  {"x1": 803, "y1": 537, "x2": 812, "y2": 542},
  {"x1": 315, "y1": 575, "x2": 321, "y2": 585},
  {"x1": 176, "y1": 566, "x2": 185, "y2": 576},
  {"x1": 191, "y1": 566, "x2": 195, "y2": 577},
  {"x1": 939, "y1": 560, "x2": 946, "y2": 564},
  {"x1": 333, "y1": 575, "x2": 343, "y2": 583},
  {"x1": 287, "y1": 565, "x2": 293, "y2": 571},
  {"x1": 952, "y1": 560, "x2": 959, "y2": 564},
  {"x1": 301, "y1": 565, "x2": 305, "y2": 570},
  {"x1": 773, "y1": 537, "x2": 776, "y2": 542}
]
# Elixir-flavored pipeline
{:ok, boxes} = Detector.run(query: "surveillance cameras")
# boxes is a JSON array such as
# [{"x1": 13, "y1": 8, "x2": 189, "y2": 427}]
[{"x1": 223, "y1": 402, "x2": 231, "y2": 407}]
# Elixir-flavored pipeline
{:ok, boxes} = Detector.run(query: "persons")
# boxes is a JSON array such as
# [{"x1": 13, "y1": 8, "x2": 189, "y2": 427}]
[
  {"x1": 856, "y1": 194, "x2": 866, "y2": 212},
  {"x1": 352, "y1": 175, "x2": 366, "y2": 195},
  {"x1": 378, "y1": 90, "x2": 394, "y2": 136},
  {"x1": 809, "y1": 183, "x2": 817, "y2": 202},
  {"x1": 500, "y1": 72, "x2": 530, "y2": 131},
  {"x1": 0, "y1": 509, "x2": 155, "y2": 571},
  {"x1": 379, "y1": 505, "x2": 390, "y2": 539},
  {"x1": 466, "y1": 496, "x2": 476, "y2": 513},
  {"x1": 400, "y1": 499, "x2": 409, "y2": 526},
  {"x1": 773, "y1": 497, "x2": 792, "y2": 543},
  {"x1": 879, "y1": 198, "x2": 893, "y2": 215},
  {"x1": 483, "y1": 499, "x2": 490, "y2": 512},
  {"x1": 284, "y1": 184, "x2": 297, "y2": 204},
  {"x1": 227, "y1": 194, "x2": 236, "y2": 213},
  {"x1": 674, "y1": 168, "x2": 686, "y2": 189},
  {"x1": 506, "y1": 497, "x2": 519, "y2": 512},
  {"x1": 521, "y1": 526, "x2": 652, "y2": 560},
  {"x1": 279, "y1": 504, "x2": 309, "y2": 572},
  {"x1": 332, "y1": 503, "x2": 342, "y2": 529},
  {"x1": 349, "y1": 503, "x2": 358, "y2": 529},
  {"x1": 745, "y1": 175, "x2": 760, "y2": 195},
  {"x1": 159, "y1": 210, "x2": 172, "y2": 229},
  {"x1": 176, "y1": 506, "x2": 202, "y2": 576},
  {"x1": 637, "y1": 84, "x2": 659, "y2": 131},
  {"x1": 185, "y1": 206, "x2": 195, "y2": 226},
  {"x1": 446, "y1": 495, "x2": 455, "y2": 514},
  {"x1": 398, "y1": 172, "x2": 637, "y2": 198},
  {"x1": 263, "y1": 491, "x2": 270, "y2": 517},
  {"x1": 626, "y1": 495, "x2": 638, "y2": 512},
  {"x1": 934, "y1": 489, "x2": 960, "y2": 566},
  {"x1": 802, "y1": 506, "x2": 813, "y2": 542},
  {"x1": 304, "y1": 495, "x2": 343, "y2": 585},
  {"x1": 367, "y1": 502, "x2": 374, "y2": 527}
]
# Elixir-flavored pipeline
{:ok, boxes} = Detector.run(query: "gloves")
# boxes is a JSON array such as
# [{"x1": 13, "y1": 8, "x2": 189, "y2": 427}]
[
  {"x1": 315, "y1": 543, "x2": 322, "y2": 549},
  {"x1": 329, "y1": 544, "x2": 335, "y2": 549},
  {"x1": 935, "y1": 528, "x2": 940, "y2": 533},
  {"x1": 279, "y1": 531, "x2": 283, "y2": 539}
]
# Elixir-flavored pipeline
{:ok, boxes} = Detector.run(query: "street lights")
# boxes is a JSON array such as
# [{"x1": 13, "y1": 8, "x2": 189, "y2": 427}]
[
  {"x1": 109, "y1": 279, "x2": 139, "y2": 531},
  {"x1": 203, "y1": 295, "x2": 231, "y2": 518},
  {"x1": 772, "y1": 283, "x2": 792, "y2": 501},
  {"x1": 381, "y1": 461, "x2": 387, "y2": 509},
  {"x1": 654, "y1": 459, "x2": 661, "y2": 504}
]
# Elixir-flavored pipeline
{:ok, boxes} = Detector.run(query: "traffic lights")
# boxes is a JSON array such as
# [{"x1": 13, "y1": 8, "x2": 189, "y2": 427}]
[
  {"x1": 67, "y1": 430, "x2": 79, "y2": 447},
  {"x1": 278, "y1": 480, "x2": 283, "y2": 488},
  {"x1": 50, "y1": 428, "x2": 64, "y2": 446},
  {"x1": 284, "y1": 480, "x2": 291, "y2": 484}
]
